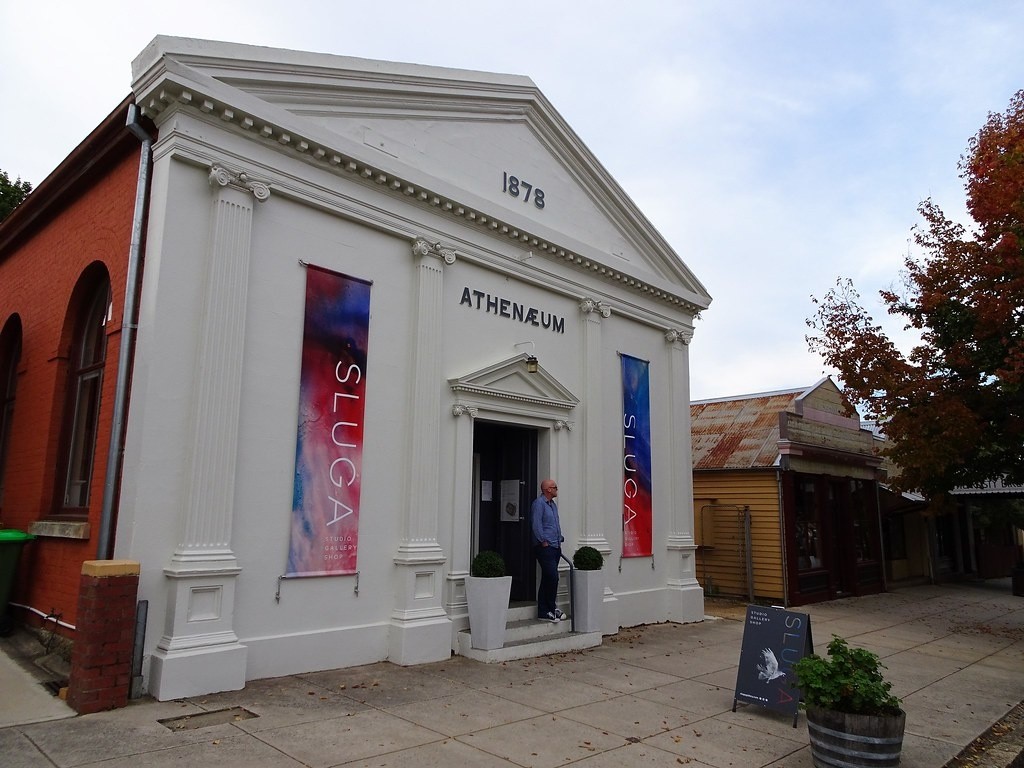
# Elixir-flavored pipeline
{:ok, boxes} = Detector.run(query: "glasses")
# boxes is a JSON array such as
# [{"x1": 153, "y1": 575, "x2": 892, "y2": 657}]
[{"x1": 550, "y1": 486, "x2": 558, "y2": 489}]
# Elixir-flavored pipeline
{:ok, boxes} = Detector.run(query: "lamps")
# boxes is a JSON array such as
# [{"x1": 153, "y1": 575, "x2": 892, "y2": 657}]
[{"x1": 514, "y1": 341, "x2": 538, "y2": 374}]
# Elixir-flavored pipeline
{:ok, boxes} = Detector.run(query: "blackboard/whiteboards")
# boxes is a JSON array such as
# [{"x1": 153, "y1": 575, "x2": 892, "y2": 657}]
[{"x1": 735, "y1": 605, "x2": 809, "y2": 715}]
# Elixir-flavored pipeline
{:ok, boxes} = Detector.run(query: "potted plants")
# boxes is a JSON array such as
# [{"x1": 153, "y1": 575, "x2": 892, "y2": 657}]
[
  {"x1": 464, "y1": 550, "x2": 512, "y2": 651},
  {"x1": 791, "y1": 633, "x2": 906, "y2": 768},
  {"x1": 567, "y1": 547, "x2": 603, "y2": 633}
]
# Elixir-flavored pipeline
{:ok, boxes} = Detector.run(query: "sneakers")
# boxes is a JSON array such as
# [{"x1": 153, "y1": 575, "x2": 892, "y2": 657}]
[
  {"x1": 537, "y1": 611, "x2": 560, "y2": 623},
  {"x1": 555, "y1": 608, "x2": 567, "y2": 620}
]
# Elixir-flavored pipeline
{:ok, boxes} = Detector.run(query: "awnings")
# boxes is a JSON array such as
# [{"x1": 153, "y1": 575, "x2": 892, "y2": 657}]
[
  {"x1": 880, "y1": 482, "x2": 932, "y2": 501},
  {"x1": 948, "y1": 468, "x2": 1024, "y2": 495}
]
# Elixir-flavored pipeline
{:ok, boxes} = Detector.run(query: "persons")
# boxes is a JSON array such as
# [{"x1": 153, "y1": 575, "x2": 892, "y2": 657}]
[{"x1": 531, "y1": 480, "x2": 567, "y2": 622}]
